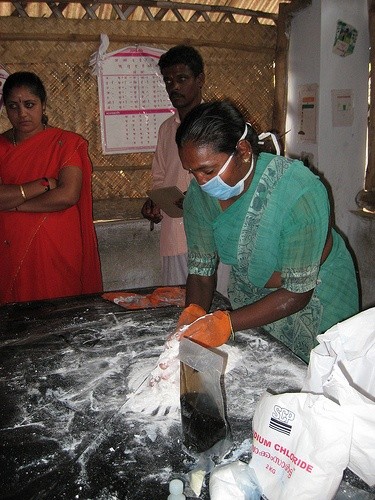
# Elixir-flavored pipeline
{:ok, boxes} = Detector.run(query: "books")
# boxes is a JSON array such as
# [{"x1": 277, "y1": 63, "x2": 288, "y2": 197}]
[{"x1": 146, "y1": 186, "x2": 185, "y2": 218}]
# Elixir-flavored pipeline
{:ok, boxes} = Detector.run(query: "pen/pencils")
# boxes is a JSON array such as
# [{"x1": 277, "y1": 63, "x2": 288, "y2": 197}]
[{"x1": 149, "y1": 199, "x2": 155, "y2": 232}]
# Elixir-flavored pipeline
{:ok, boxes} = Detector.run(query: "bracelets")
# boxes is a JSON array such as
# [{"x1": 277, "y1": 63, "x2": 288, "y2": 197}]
[
  {"x1": 43, "y1": 177, "x2": 50, "y2": 191},
  {"x1": 228, "y1": 311, "x2": 235, "y2": 341},
  {"x1": 19, "y1": 184, "x2": 27, "y2": 202}
]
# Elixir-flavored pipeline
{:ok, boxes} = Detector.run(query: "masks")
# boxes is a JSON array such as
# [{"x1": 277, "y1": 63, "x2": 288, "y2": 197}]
[{"x1": 193, "y1": 123, "x2": 281, "y2": 201}]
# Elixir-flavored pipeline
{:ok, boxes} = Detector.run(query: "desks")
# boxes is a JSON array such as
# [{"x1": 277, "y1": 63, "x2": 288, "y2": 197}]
[{"x1": 1, "y1": 284, "x2": 375, "y2": 500}]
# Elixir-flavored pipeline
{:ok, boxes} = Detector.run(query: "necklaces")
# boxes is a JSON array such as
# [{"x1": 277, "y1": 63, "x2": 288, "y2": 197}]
[{"x1": 12, "y1": 125, "x2": 46, "y2": 150}]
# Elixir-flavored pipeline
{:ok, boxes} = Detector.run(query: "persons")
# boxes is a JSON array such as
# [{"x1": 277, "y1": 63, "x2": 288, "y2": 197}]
[
  {"x1": 0, "y1": 72, "x2": 103, "y2": 303},
  {"x1": 141, "y1": 44, "x2": 232, "y2": 299},
  {"x1": 149, "y1": 100, "x2": 359, "y2": 388}
]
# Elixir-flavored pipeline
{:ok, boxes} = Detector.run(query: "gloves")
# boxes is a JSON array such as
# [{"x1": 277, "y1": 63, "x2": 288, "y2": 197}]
[{"x1": 148, "y1": 304, "x2": 234, "y2": 386}]
